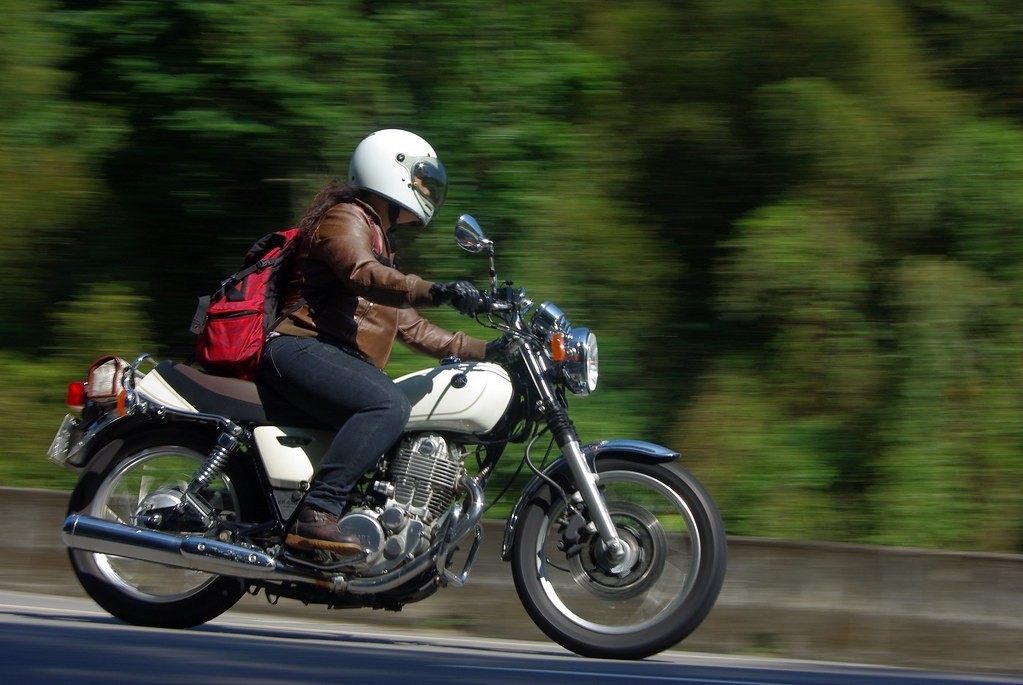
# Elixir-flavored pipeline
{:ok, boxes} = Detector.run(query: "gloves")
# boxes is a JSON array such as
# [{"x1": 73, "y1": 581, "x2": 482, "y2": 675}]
[
  {"x1": 485, "y1": 337, "x2": 513, "y2": 364},
  {"x1": 428, "y1": 281, "x2": 481, "y2": 318}
]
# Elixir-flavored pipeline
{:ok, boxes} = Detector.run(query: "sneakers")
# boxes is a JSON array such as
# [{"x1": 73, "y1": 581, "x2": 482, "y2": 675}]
[{"x1": 286, "y1": 505, "x2": 362, "y2": 556}]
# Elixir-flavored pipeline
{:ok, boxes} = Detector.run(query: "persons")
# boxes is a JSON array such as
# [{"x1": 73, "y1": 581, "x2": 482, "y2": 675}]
[{"x1": 260, "y1": 128, "x2": 513, "y2": 555}]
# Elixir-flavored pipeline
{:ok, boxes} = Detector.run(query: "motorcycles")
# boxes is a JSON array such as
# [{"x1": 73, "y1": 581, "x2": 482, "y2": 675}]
[{"x1": 44, "y1": 213, "x2": 725, "y2": 659}]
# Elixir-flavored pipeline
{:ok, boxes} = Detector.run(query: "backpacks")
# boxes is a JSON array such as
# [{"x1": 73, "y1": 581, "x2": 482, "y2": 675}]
[{"x1": 189, "y1": 201, "x2": 382, "y2": 380}]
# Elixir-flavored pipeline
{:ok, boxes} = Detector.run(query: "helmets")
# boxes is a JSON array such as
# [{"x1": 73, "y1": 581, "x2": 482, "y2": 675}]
[{"x1": 347, "y1": 128, "x2": 447, "y2": 232}]
